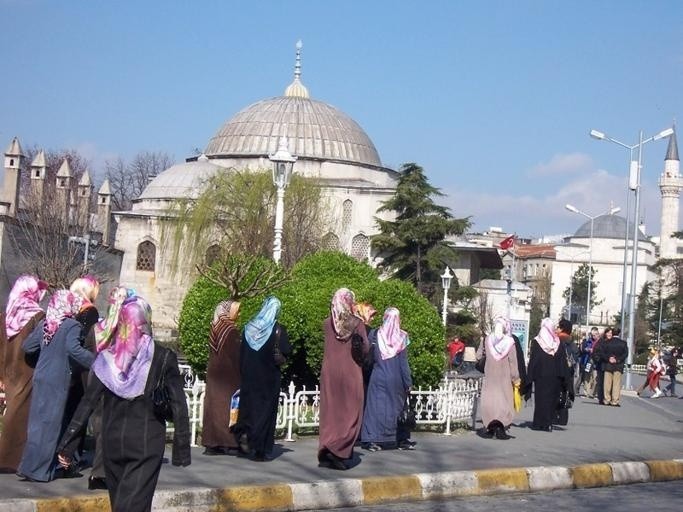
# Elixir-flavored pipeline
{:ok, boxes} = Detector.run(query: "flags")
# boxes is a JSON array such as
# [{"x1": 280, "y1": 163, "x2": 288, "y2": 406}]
[{"x1": 500, "y1": 235, "x2": 514, "y2": 250}]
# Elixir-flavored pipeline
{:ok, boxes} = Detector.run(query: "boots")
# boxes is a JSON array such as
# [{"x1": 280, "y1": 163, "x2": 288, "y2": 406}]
[{"x1": 655, "y1": 387, "x2": 661, "y2": 397}]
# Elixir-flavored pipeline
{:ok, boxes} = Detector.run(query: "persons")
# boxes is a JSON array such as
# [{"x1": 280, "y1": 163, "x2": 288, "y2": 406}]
[
  {"x1": 353, "y1": 301, "x2": 378, "y2": 442},
  {"x1": 360, "y1": 306, "x2": 417, "y2": 451},
  {"x1": 79, "y1": 286, "x2": 140, "y2": 488},
  {"x1": 318, "y1": 288, "x2": 369, "y2": 469},
  {"x1": 447, "y1": 316, "x2": 681, "y2": 442},
  {"x1": 57, "y1": 297, "x2": 191, "y2": 511},
  {"x1": 0, "y1": 275, "x2": 50, "y2": 472},
  {"x1": 201, "y1": 300, "x2": 243, "y2": 457},
  {"x1": 17, "y1": 290, "x2": 99, "y2": 481},
  {"x1": 66, "y1": 276, "x2": 100, "y2": 467},
  {"x1": 236, "y1": 297, "x2": 293, "y2": 460}
]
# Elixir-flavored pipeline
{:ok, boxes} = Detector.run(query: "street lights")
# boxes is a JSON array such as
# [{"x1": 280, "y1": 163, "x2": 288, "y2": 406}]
[
  {"x1": 440, "y1": 265, "x2": 454, "y2": 325},
  {"x1": 565, "y1": 203, "x2": 622, "y2": 335},
  {"x1": 590, "y1": 129, "x2": 674, "y2": 389},
  {"x1": 267, "y1": 133, "x2": 298, "y2": 276}
]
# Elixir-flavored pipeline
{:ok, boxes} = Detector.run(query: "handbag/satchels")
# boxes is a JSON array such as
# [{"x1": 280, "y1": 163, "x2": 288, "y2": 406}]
[
  {"x1": 560, "y1": 391, "x2": 572, "y2": 408},
  {"x1": 274, "y1": 344, "x2": 286, "y2": 365},
  {"x1": 352, "y1": 333, "x2": 366, "y2": 367},
  {"x1": 398, "y1": 392, "x2": 416, "y2": 428},
  {"x1": 153, "y1": 384, "x2": 173, "y2": 422},
  {"x1": 514, "y1": 385, "x2": 522, "y2": 412}
]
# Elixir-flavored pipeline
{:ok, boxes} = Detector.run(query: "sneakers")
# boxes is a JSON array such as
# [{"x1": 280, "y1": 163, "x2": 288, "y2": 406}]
[
  {"x1": 398, "y1": 440, "x2": 416, "y2": 450},
  {"x1": 362, "y1": 443, "x2": 380, "y2": 451}
]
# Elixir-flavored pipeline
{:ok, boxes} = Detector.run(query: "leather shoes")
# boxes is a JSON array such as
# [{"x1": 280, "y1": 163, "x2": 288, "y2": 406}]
[{"x1": 89, "y1": 476, "x2": 107, "y2": 489}]
